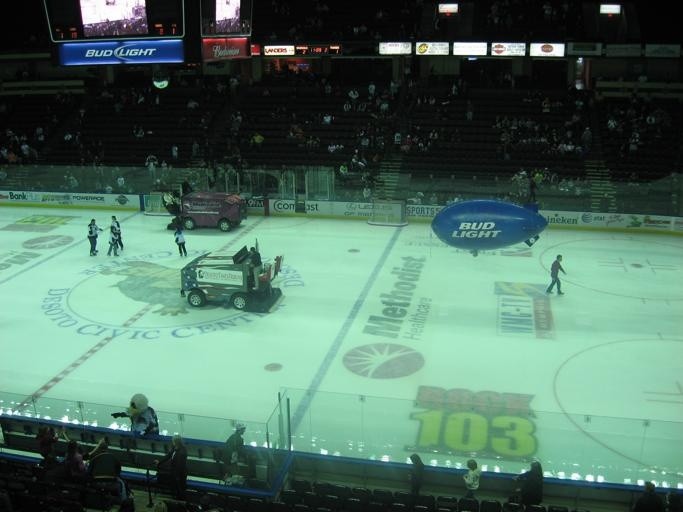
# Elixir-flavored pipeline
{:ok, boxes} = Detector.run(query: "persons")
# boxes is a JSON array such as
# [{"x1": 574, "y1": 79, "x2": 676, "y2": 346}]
[
  {"x1": 0, "y1": 81, "x2": 683, "y2": 206},
  {"x1": 635, "y1": 481, "x2": 667, "y2": 512},
  {"x1": 34, "y1": 394, "x2": 259, "y2": 489},
  {"x1": 250, "y1": 247, "x2": 262, "y2": 268},
  {"x1": 174, "y1": 225, "x2": 188, "y2": 257},
  {"x1": 518, "y1": 462, "x2": 544, "y2": 505},
  {"x1": 88, "y1": 219, "x2": 103, "y2": 257},
  {"x1": 463, "y1": 461, "x2": 482, "y2": 499},
  {"x1": 545, "y1": 255, "x2": 567, "y2": 295},
  {"x1": 409, "y1": 453, "x2": 423, "y2": 495},
  {"x1": 108, "y1": 225, "x2": 120, "y2": 257},
  {"x1": 111, "y1": 215, "x2": 123, "y2": 250}
]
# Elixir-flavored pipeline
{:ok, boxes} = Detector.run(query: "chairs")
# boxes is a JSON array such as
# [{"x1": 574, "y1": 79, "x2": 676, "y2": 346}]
[{"x1": 0, "y1": 461, "x2": 570, "y2": 512}]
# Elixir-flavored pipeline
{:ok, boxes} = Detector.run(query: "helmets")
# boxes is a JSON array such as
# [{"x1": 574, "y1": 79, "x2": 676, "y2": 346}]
[{"x1": 127, "y1": 394, "x2": 149, "y2": 416}]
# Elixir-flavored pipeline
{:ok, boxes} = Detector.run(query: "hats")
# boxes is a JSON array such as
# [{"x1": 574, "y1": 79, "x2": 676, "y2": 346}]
[{"x1": 235, "y1": 422, "x2": 246, "y2": 431}]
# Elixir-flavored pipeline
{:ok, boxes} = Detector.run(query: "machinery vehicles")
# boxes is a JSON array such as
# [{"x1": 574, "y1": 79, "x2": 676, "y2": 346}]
[
  {"x1": 161, "y1": 181, "x2": 248, "y2": 232},
  {"x1": 180, "y1": 237, "x2": 286, "y2": 314}
]
